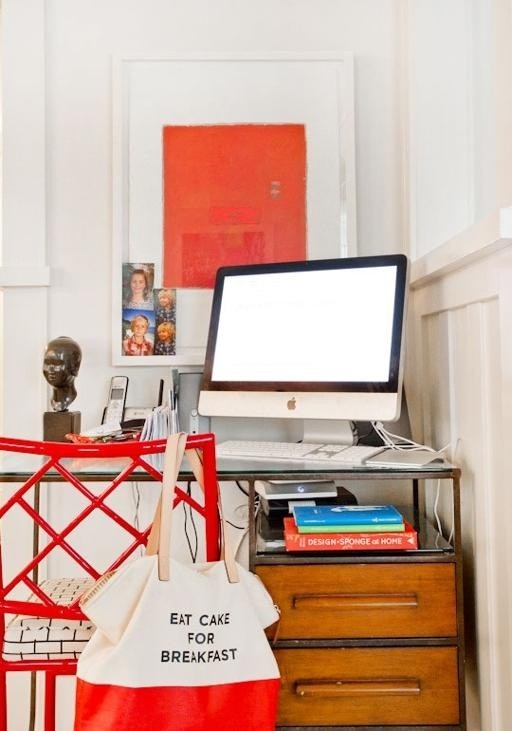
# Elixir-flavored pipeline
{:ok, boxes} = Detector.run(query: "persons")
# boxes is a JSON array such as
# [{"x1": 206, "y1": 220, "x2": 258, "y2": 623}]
[
  {"x1": 154, "y1": 288, "x2": 175, "y2": 320},
  {"x1": 122, "y1": 314, "x2": 153, "y2": 356},
  {"x1": 154, "y1": 321, "x2": 175, "y2": 354},
  {"x1": 123, "y1": 268, "x2": 153, "y2": 309},
  {"x1": 42, "y1": 336, "x2": 83, "y2": 410}
]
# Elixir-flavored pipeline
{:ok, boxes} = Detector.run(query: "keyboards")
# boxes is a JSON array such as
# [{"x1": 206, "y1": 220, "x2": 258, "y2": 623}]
[{"x1": 202, "y1": 441, "x2": 385, "y2": 464}]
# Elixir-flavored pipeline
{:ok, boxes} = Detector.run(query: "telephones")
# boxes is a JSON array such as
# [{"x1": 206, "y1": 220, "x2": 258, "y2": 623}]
[{"x1": 101, "y1": 376, "x2": 164, "y2": 429}]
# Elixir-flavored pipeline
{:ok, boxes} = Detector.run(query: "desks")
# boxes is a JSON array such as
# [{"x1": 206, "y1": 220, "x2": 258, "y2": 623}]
[{"x1": 0, "y1": 448, "x2": 468, "y2": 731}]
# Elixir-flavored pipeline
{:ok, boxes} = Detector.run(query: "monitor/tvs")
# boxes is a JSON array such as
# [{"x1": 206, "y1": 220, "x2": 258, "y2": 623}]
[{"x1": 197, "y1": 253, "x2": 411, "y2": 445}]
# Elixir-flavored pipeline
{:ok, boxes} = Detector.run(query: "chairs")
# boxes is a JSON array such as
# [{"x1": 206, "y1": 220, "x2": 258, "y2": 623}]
[{"x1": 0, "y1": 434, "x2": 220, "y2": 731}]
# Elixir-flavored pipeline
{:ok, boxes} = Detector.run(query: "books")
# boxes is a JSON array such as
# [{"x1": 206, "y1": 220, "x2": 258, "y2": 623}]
[
  {"x1": 292, "y1": 504, "x2": 403, "y2": 524},
  {"x1": 282, "y1": 517, "x2": 418, "y2": 551},
  {"x1": 296, "y1": 522, "x2": 406, "y2": 532}
]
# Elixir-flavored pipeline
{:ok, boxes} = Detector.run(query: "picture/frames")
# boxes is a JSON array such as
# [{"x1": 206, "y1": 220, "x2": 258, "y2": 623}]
[{"x1": 111, "y1": 47, "x2": 360, "y2": 370}]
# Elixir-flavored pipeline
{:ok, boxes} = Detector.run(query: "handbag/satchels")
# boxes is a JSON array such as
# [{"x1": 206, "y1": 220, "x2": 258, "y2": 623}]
[{"x1": 72, "y1": 429, "x2": 282, "y2": 731}]
